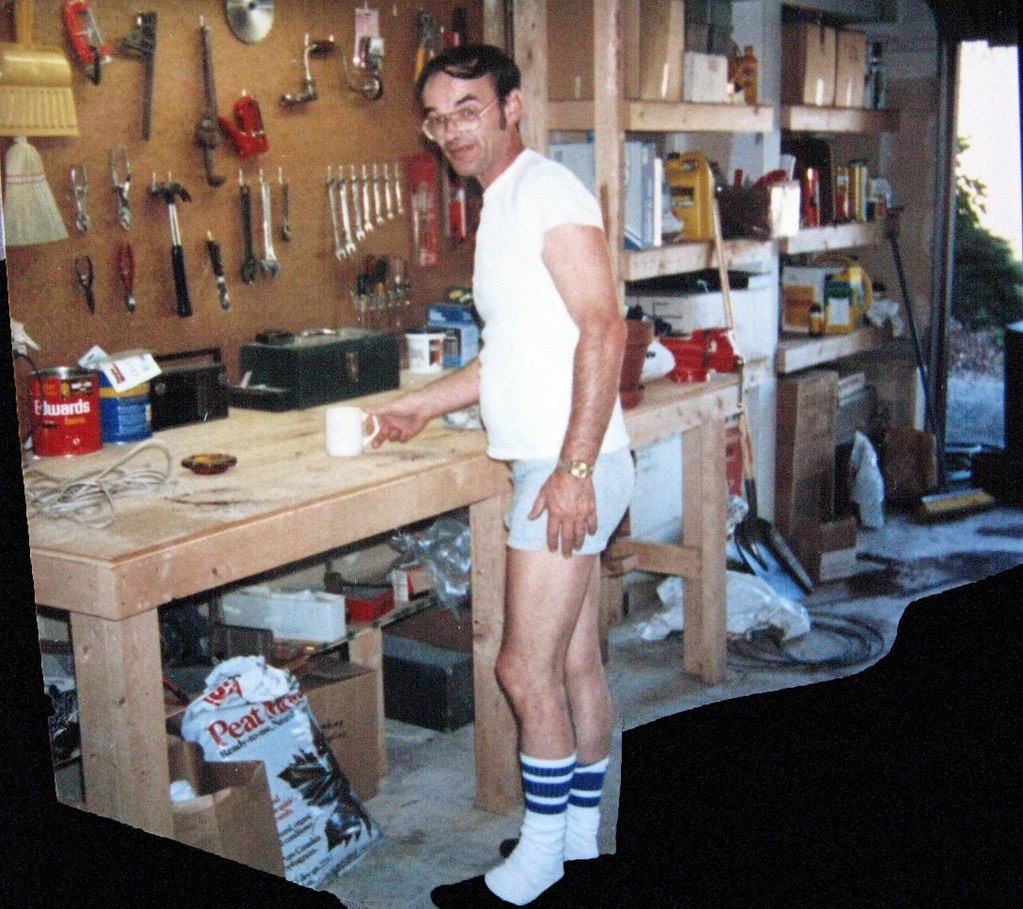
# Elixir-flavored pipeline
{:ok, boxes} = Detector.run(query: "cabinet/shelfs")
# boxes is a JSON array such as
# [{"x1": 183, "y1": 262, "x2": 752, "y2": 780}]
[
  {"x1": 512, "y1": 0, "x2": 771, "y2": 451},
  {"x1": 771, "y1": 0, "x2": 905, "y2": 377}
]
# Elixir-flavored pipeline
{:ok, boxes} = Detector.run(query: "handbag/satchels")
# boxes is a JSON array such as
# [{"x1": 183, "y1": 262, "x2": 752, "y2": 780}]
[{"x1": 182, "y1": 654, "x2": 382, "y2": 891}]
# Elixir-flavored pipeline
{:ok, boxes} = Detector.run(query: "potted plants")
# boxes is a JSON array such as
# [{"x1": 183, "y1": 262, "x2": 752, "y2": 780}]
[{"x1": 618, "y1": 305, "x2": 654, "y2": 409}]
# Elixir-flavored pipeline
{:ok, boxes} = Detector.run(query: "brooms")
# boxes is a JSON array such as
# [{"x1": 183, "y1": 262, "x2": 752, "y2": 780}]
[
  {"x1": 3, "y1": 136, "x2": 69, "y2": 248},
  {"x1": 885, "y1": 227, "x2": 996, "y2": 521}
]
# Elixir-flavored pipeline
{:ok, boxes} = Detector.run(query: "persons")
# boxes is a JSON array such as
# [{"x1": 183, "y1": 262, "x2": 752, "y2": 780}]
[{"x1": 363, "y1": 46, "x2": 638, "y2": 909}]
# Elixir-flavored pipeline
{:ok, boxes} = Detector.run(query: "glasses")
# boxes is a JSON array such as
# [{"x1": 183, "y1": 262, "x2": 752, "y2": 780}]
[{"x1": 421, "y1": 98, "x2": 501, "y2": 140}]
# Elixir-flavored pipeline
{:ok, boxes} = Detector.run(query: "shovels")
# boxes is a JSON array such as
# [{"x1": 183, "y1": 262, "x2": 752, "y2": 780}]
[{"x1": 709, "y1": 198, "x2": 815, "y2": 601}]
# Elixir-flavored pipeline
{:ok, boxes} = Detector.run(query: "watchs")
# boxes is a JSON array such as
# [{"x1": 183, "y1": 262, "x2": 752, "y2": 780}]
[{"x1": 556, "y1": 460, "x2": 596, "y2": 479}]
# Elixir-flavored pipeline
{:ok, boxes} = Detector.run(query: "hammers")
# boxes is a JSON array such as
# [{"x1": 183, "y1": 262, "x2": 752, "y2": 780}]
[{"x1": 146, "y1": 180, "x2": 193, "y2": 318}]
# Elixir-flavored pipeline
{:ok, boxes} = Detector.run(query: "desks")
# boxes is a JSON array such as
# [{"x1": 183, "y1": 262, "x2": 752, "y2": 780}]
[{"x1": 19, "y1": 366, "x2": 745, "y2": 841}]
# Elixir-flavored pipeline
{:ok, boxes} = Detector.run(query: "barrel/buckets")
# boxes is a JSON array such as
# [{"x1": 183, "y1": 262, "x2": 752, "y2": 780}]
[
  {"x1": 668, "y1": 151, "x2": 717, "y2": 243},
  {"x1": 822, "y1": 255, "x2": 872, "y2": 335},
  {"x1": 27, "y1": 361, "x2": 153, "y2": 457}
]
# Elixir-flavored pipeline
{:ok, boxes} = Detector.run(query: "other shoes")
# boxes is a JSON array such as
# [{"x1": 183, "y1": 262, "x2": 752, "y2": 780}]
[
  {"x1": 431, "y1": 873, "x2": 569, "y2": 909},
  {"x1": 500, "y1": 838, "x2": 602, "y2": 874}
]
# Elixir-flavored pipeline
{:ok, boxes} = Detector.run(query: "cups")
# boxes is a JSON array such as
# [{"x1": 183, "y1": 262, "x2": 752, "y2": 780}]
[{"x1": 325, "y1": 406, "x2": 379, "y2": 457}]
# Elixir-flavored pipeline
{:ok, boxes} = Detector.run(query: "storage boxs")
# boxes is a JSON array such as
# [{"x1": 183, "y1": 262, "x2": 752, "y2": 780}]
[
  {"x1": 773, "y1": 357, "x2": 947, "y2": 585},
  {"x1": 166, "y1": 654, "x2": 381, "y2": 893},
  {"x1": 228, "y1": 326, "x2": 401, "y2": 413}
]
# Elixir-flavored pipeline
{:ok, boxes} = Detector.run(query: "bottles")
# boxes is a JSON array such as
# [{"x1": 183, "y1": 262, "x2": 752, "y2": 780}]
[
  {"x1": 802, "y1": 162, "x2": 890, "y2": 227},
  {"x1": 740, "y1": 45, "x2": 757, "y2": 105}
]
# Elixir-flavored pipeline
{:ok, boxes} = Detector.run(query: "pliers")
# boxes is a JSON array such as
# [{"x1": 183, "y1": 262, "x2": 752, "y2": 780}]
[
  {"x1": 73, "y1": 254, "x2": 97, "y2": 313},
  {"x1": 109, "y1": 145, "x2": 132, "y2": 232},
  {"x1": 68, "y1": 164, "x2": 93, "y2": 235},
  {"x1": 117, "y1": 245, "x2": 136, "y2": 313}
]
